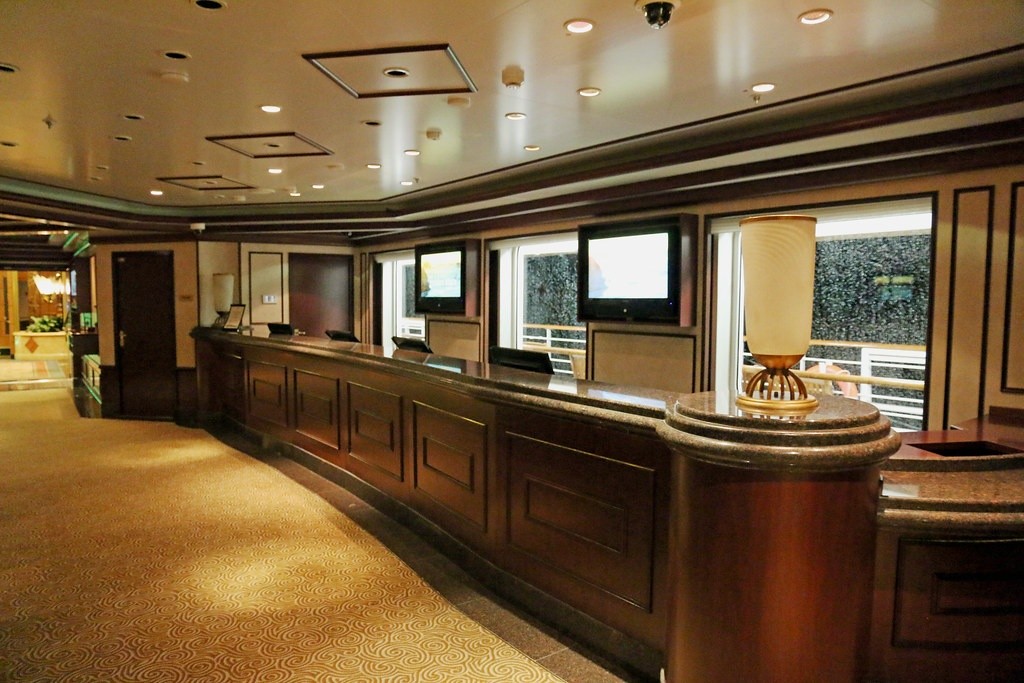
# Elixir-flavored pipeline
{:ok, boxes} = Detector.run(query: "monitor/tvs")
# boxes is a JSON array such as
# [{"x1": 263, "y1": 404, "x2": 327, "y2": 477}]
[
  {"x1": 490, "y1": 346, "x2": 555, "y2": 374},
  {"x1": 326, "y1": 330, "x2": 360, "y2": 343},
  {"x1": 268, "y1": 323, "x2": 294, "y2": 335},
  {"x1": 392, "y1": 336, "x2": 433, "y2": 354}
]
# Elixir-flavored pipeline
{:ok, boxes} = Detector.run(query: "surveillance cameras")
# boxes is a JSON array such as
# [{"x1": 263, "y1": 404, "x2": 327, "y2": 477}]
[{"x1": 642, "y1": 3, "x2": 674, "y2": 29}]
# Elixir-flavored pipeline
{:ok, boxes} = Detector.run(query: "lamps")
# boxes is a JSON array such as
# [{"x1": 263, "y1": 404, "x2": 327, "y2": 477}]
[
  {"x1": 211, "y1": 274, "x2": 234, "y2": 327},
  {"x1": 737, "y1": 215, "x2": 819, "y2": 409}
]
